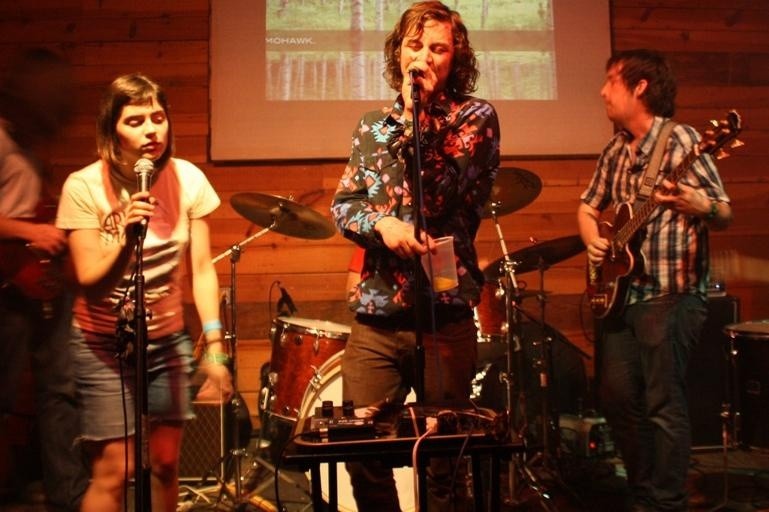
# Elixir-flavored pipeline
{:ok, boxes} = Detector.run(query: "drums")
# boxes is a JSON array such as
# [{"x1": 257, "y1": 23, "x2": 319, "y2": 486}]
[
  {"x1": 260, "y1": 314, "x2": 352, "y2": 424},
  {"x1": 469, "y1": 276, "x2": 511, "y2": 367},
  {"x1": 290, "y1": 351, "x2": 424, "y2": 512}
]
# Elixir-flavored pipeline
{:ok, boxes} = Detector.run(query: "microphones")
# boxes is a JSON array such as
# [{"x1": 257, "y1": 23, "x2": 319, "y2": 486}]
[
  {"x1": 274, "y1": 280, "x2": 300, "y2": 318},
  {"x1": 133, "y1": 158, "x2": 155, "y2": 238},
  {"x1": 408, "y1": 67, "x2": 424, "y2": 107}
]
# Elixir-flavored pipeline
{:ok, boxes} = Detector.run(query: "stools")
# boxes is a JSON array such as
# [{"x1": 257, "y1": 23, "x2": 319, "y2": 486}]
[{"x1": 722, "y1": 321, "x2": 769, "y2": 453}]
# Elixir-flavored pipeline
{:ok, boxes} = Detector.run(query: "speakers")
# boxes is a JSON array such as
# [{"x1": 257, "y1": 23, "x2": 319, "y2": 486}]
[
  {"x1": 177, "y1": 395, "x2": 233, "y2": 484},
  {"x1": 688, "y1": 295, "x2": 741, "y2": 454}
]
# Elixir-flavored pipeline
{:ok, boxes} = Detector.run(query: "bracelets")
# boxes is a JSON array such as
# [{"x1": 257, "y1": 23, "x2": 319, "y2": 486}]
[
  {"x1": 202, "y1": 351, "x2": 230, "y2": 366},
  {"x1": 206, "y1": 338, "x2": 224, "y2": 344},
  {"x1": 707, "y1": 201, "x2": 717, "y2": 221},
  {"x1": 201, "y1": 320, "x2": 223, "y2": 333}
]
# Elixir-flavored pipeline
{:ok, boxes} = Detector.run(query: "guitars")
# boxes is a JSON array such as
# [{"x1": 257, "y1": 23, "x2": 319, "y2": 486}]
[
  {"x1": 583, "y1": 110, "x2": 745, "y2": 322},
  {"x1": 0, "y1": 211, "x2": 69, "y2": 313}
]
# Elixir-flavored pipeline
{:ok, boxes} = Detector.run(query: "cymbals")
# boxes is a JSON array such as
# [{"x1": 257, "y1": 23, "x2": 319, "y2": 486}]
[
  {"x1": 481, "y1": 231, "x2": 586, "y2": 280},
  {"x1": 229, "y1": 193, "x2": 337, "y2": 239},
  {"x1": 481, "y1": 166, "x2": 542, "y2": 220}
]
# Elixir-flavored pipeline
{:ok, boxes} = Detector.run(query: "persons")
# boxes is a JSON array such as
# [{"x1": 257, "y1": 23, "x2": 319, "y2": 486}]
[
  {"x1": 1, "y1": 113, "x2": 68, "y2": 511},
  {"x1": 577, "y1": 50, "x2": 733, "y2": 511},
  {"x1": 54, "y1": 73, "x2": 233, "y2": 511},
  {"x1": 330, "y1": 0, "x2": 500, "y2": 511}
]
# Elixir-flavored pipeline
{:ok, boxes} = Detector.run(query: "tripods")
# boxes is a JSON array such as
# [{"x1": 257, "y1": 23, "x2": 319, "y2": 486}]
[{"x1": 184, "y1": 214, "x2": 326, "y2": 512}]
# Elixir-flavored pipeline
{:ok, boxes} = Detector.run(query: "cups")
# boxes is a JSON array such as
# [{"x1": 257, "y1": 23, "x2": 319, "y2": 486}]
[{"x1": 419, "y1": 234, "x2": 460, "y2": 293}]
